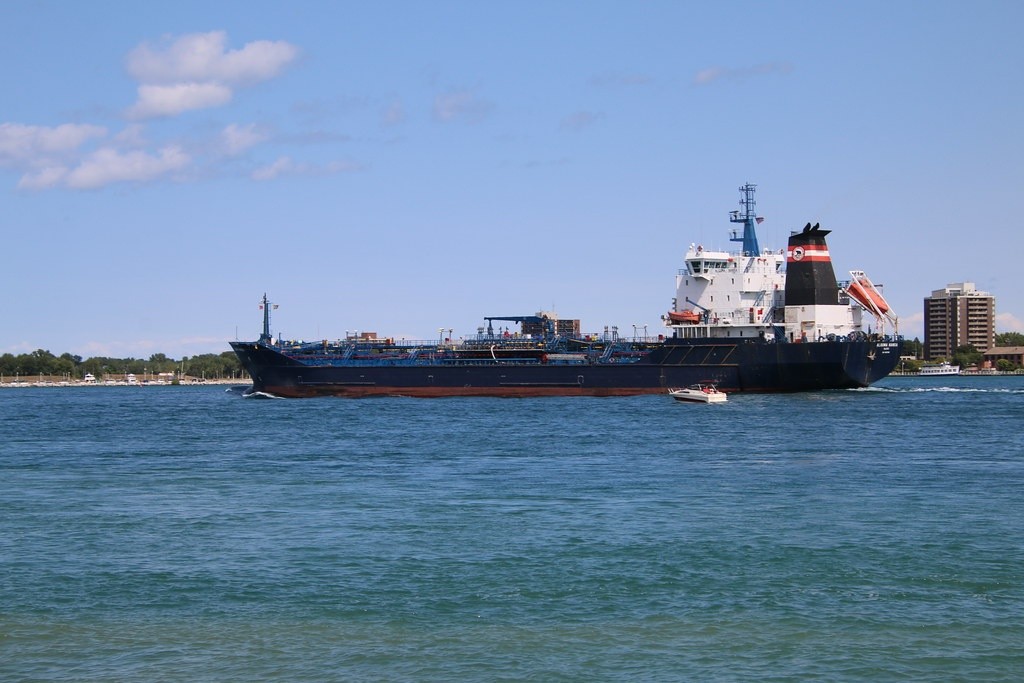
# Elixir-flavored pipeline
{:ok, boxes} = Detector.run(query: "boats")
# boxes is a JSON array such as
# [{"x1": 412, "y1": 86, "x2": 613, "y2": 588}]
[
  {"x1": 666, "y1": 376, "x2": 729, "y2": 404},
  {"x1": 668, "y1": 310, "x2": 703, "y2": 325},
  {"x1": 918, "y1": 360, "x2": 961, "y2": 376}
]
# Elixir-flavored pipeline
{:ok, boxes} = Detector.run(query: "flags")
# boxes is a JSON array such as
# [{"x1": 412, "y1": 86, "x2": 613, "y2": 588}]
[{"x1": 756, "y1": 217, "x2": 764, "y2": 224}]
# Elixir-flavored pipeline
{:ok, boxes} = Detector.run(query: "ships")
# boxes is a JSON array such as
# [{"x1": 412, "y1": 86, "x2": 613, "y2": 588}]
[{"x1": 226, "y1": 181, "x2": 905, "y2": 400}]
